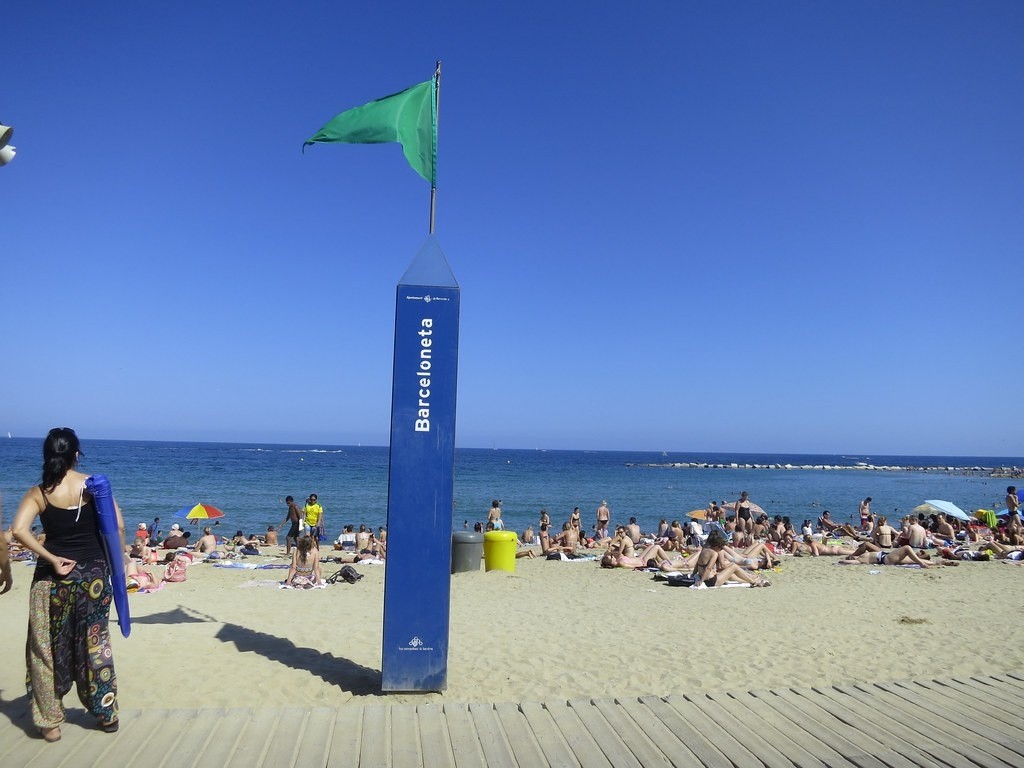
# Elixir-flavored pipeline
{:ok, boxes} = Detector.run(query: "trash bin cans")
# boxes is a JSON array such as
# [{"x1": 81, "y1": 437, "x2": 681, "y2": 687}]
[
  {"x1": 451, "y1": 531, "x2": 484, "y2": 574},
  {"x1": 484, "y1": 530, "x2": 518, "y2": 573}
]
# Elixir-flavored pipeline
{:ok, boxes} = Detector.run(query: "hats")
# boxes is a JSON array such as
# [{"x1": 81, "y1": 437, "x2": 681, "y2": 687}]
[
  {"x1": 306, "y1": 496, "x2": 310, "y2": 502},
  {"x1": 166, "y1": 552, "x2": 175, "y2": 561},
  {"x1": 139, "y1": 522, "x2": 146, "y2": 529},
  {"x1": 172, "y1": 524, "x2": 179, "y2": 529}
]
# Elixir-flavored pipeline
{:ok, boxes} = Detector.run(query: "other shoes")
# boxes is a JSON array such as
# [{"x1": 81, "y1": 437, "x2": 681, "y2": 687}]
[
  {"x1": 751, "y1": 579, "x2": 771, "y2": 588},
  {"x1": 35, "y1": 725, "x2": 62, "y2": 743},
  {"x1": 101, "y1": 717, "x2": 119, "y2": 733}
]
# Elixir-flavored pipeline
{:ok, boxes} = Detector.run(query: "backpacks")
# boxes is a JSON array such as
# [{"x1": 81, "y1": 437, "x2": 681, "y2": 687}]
[{"x1": 148, "y1": 524, "x2": 157, "y2": 534}]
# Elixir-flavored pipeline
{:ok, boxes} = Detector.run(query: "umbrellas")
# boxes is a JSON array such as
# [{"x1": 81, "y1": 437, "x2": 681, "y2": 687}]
[
  {"x1": 997, "y1": 490, "x2": 1024, "y2": 526},
  {"x1": 685, "y1": 505, "x2": 716, "y2": 524},
  {"x1": 925, "y1": 497, "x2": 971, "y2": 524},
  {"x1": 173, "y1": 502, "x2": 225, "y2": 541},
  {"x1": 723, "y1": 497, "x2": 768, "y2": 518}
]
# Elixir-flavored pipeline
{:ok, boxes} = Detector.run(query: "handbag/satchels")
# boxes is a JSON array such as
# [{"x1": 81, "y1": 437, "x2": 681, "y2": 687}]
[
  {"x1": 334, "y1": 544, "x2": 342, "y2": 550},
  {"x1": 326, "y1": 565, "x2": 364, "y2": 585},
  {"x1": 299, "y1": 519, "x2": 305, "y2": 532},
  {"x1": 318, "y1": 524, "x2": 327, "y2": 541},
  {"x1": 208, "y1": 551, "x2": 227, "y2": 560},
  {"x1": 668, "y1": 574, "x2": 695, "y2": 586},
  {"x1": 164, "y1": 558, "x2": 187, "y2": 582},
  {"x1": 241, "y1": 544, "x2": 259, "y2": 555}
]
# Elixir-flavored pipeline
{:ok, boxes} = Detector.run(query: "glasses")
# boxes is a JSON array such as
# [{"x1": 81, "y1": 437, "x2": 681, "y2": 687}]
[
  {"x1": 617, "y1": 531, "x2": 625, "y2": 534},
  {"x1": 310, "y1": 499, "x2": 316, "y2": 501},
  {"x1": 49, "y1": 427, "x2": 75, "y2": 433},
  {"x1": 609, "y1": 555, "x2": 614, "y2": 559}
]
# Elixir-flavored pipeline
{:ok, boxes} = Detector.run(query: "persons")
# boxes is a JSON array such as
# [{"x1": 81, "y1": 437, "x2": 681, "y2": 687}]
[
  {"x1": 0, "y1": 525, "x2": 46, "y2": 595},
  {"x1": 463, "y1": 485, "x2": 1024, "y2": 587},
  {"x1": 194, "y1": 494, "x2": 386, "y2": 589},
  {"x1": 15, "y1": 425, "x2": 126, "y2": 741},
  {"x1": 125, "y1": 517, "x2": 192, "y2": 592}
]
